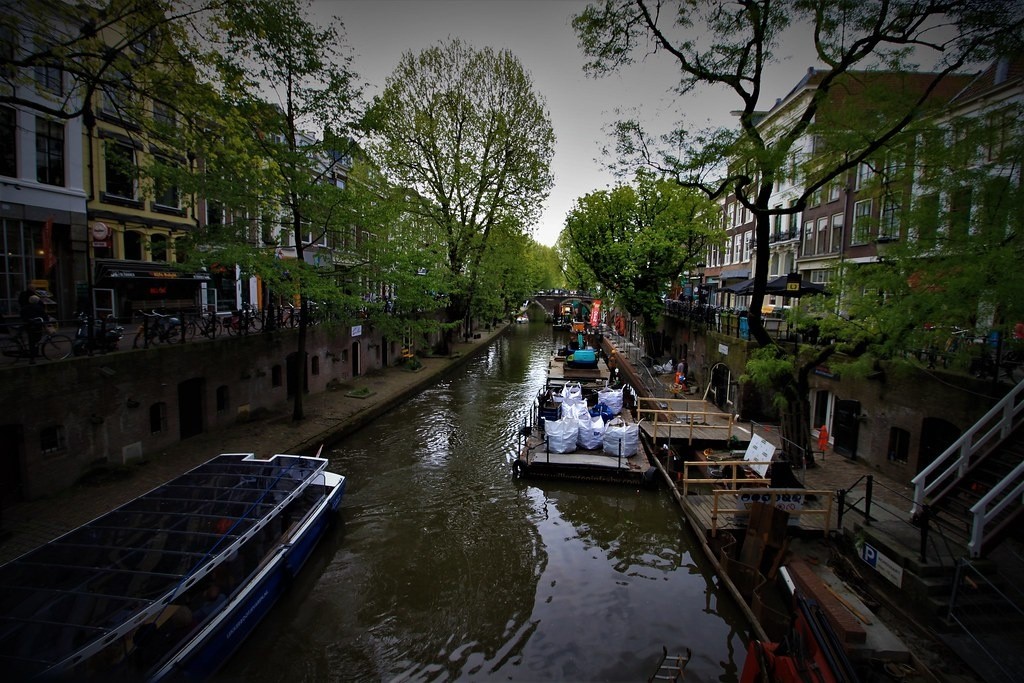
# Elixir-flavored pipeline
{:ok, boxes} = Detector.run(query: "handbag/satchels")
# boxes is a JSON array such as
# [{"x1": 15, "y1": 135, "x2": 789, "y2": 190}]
[
  {"x1": 589, "y1": 401, "x2": 614, "y2": 424},
  {"x1": 597, "y1": 381, "x2": 627, "y2": 415},
  {"x1": 561, "y1": 381, "x2": 582, "y2": 401},
  {"x1": 602, "y1": 415, "x2": 644, "y2": 457},
  {"x1": 541, "y1": 414, "x2": 578, "y2": 453},
  {"x1": 561, "y1": 398, "x2": 592, "y2": 420},
  {"x1": 576, "y1": 409, "x2": 605, "y2": 450}
]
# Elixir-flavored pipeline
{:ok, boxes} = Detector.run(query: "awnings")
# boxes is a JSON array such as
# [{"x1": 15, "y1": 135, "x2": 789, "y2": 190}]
[{"x1": 94, "y1": 260, "x2": 212, "y2": 285}]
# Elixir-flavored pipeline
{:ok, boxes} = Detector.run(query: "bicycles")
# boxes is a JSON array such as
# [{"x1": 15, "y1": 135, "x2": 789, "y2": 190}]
[
  {"x1": 0, "y1": 322, "x2": 72, "y2": 362},
  {"x1": 132, "y1": 296, "x2": 325, "y2": 350}
]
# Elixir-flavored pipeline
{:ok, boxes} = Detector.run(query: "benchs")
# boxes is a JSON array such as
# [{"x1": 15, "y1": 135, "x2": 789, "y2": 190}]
[{"x1": 762, "y1": 305, "x2": 786, "y2": 317}]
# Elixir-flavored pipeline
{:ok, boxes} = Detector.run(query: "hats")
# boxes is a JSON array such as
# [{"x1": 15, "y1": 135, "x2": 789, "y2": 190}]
[{"x1": 681, "y1": 359, "x2": 686, "y2": 363}]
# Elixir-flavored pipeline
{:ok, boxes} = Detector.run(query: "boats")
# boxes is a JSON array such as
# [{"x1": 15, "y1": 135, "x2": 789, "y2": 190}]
[
  {"x1": 511, "y1": 323, "x2": 653, "y2": 487},
  {"x1": 0, "y1": 442, "x2": 347, "y2": 683}
]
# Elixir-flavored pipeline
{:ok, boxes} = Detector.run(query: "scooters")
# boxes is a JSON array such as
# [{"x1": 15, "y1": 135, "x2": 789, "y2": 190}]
[{"x1": 72, "y1": 314, "x2": 125, "y2": 357}]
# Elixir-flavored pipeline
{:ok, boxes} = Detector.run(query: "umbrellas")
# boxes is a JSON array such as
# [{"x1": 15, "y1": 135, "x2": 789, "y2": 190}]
[
  {"x1": 714, "y1": 276, "x2": 768, "y2": 296},
  {"x1": 748, "y1": 273, "x2": 834, "y2": 307},
  {"x1": 817, "y1": 425, "x2": 829, "y2": 460}
]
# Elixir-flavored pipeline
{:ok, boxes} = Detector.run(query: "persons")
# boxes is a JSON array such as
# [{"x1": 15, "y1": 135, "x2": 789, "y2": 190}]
[
  {"x1": 492, "y1": 314, "x2": 497, "y2": 330},
  {"x1": 18, "y1": 283, "x2": 44, "y2": 355},
  {"x1": 675, "y1": 359, "x2": 689, "y2": 393},
  {"x1": 607, "y1": 350, "x2": 617, "y2": 383},
  {"x1": 569, "y1": 337, "x2": 579, "y2": 349},
  {"x1": 192, "y1": 586, "x2": 226, "y2": 619},
  {"x1": 679, "y1": 288, "x2": 695, "y2": 304}
]
